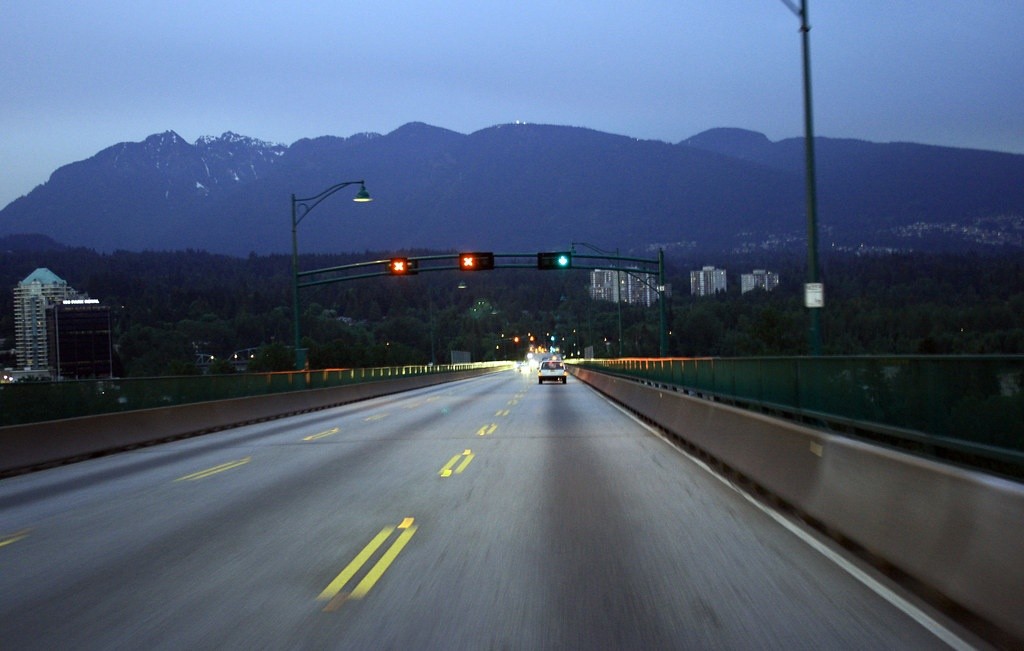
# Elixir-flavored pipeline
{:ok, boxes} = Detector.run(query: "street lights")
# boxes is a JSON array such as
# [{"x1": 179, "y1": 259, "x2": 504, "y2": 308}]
[
  {"x1": 428, "y1": 274, "x2": 468, "y2": 366},
  {"x1": 568, "y1": 242, "x2": 624, "y2": 359},
  {"x1": 291, "y1": 178, "x2": 374, "y2": 346}
]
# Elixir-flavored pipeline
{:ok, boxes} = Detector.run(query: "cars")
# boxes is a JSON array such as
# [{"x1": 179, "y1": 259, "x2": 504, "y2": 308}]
[{"x1": 537, "y1": 360, "x2": 566, "y2": 384}]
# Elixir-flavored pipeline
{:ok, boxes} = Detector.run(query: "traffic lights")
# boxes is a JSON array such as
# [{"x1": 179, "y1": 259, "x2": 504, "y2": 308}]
[
  {"x1": 390, "y1": 257, "x2": 409, "y2": 274},
  {"x1": 537, "y1": 252, "x2": 572, "y2": 270},
  {"x1": 458, "y1": 252, "x2": 494, "y2": 272}
]
